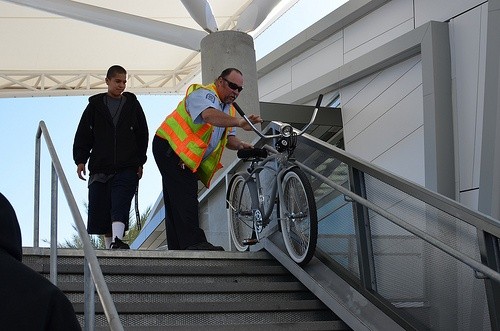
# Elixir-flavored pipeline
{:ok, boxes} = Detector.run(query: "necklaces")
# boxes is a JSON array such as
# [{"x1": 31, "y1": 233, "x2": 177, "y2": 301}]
[{"x1": 106, "y1": 94, "x2": 122, "y2": 120}]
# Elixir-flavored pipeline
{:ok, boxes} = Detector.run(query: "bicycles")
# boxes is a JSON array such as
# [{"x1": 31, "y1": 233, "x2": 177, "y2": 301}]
[{"x1": 224, "y1": 94, "x2": 324, "y2": 266}]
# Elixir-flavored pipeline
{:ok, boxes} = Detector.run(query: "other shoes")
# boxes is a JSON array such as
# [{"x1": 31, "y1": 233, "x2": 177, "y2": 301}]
[
  {"x1": 109, "y1": 236, "x2": 130, "y2": 249},
  {"x1": 187, "y1": 241, "x2": 224, "y2": 251}
]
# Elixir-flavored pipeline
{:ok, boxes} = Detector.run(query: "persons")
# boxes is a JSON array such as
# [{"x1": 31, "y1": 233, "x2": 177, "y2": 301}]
[
  {"x1": 72, "y1": 64, "x2": 149, "y2": 250},
  {"x1": 153, "y1": 67, "x2": 264, "y2": 251},
  {"x1": 0, "y1": 192, "x2": 82, "y2": 331}
]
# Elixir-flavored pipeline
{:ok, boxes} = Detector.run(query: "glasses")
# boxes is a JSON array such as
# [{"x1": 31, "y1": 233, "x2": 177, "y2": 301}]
[{"x1": 221, "y1": 77, "x2": 243, "y2": 93}]
